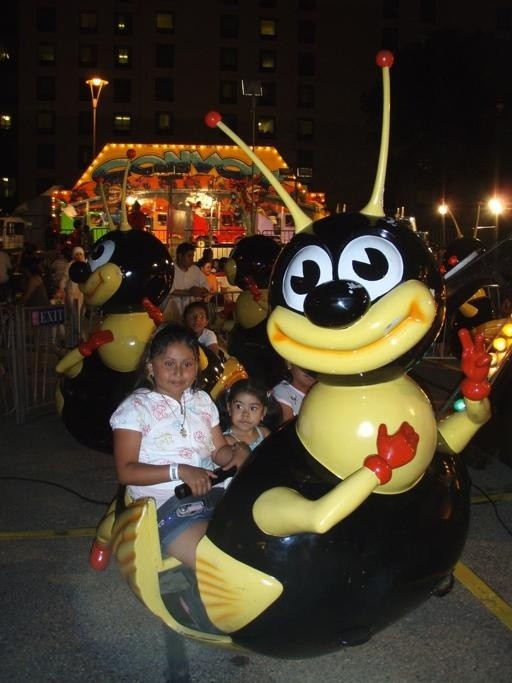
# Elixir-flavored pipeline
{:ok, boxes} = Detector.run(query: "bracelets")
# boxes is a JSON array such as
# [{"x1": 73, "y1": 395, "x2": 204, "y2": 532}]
[{"x1": 169, "y1": 463, "x2": 180, "y2": 481}]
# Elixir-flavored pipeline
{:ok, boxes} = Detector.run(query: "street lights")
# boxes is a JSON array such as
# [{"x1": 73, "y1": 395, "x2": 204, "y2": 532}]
[
  {"x1": 437, "y1": 200, "x2": 447, "y2": 246},
  {"x1": 242, "y1": 79, "x2": 262, "y2": 235},
  {"x1": 491, "y1": 197, "x2": 501, "y2": 240},
  {"x1": 85, "y1": 77, "x2": 109, "y2": 159}
]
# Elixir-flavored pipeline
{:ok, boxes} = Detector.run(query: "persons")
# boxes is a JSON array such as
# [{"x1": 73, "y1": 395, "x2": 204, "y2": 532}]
[
  {"x1": 106, "y1": 319, "x2": 251, "y2": 635},
  {"x1": 222, "y1": 377, "x2": 283, "y2": 490},
  {"x1": 183, "y1": 301, "x2": 220, "y2": 356},
  {"x1": 0, "y1": 200, "x2": 232, "y2": 349},
  {"x1": 270, "y1": 358, "x2": 318, "y2": 427}
]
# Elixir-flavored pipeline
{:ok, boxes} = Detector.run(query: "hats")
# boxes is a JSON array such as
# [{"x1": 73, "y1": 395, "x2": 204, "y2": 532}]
[{"x1": 71, "y1": 247, "x2": 84, "y2": 257}]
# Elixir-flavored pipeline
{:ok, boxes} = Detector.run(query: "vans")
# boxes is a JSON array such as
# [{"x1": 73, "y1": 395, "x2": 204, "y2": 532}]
[{"x1": 1, "y1": 217, "x2": 27, "y2": 250}]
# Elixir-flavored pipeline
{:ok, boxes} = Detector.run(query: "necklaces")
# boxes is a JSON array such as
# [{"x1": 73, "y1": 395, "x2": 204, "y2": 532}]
[{"x1": 159, "y1": 390, "x2": 187, "y2": 437}]
